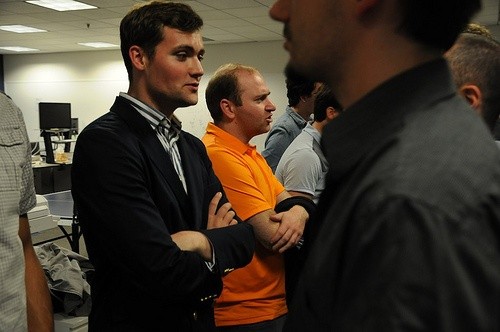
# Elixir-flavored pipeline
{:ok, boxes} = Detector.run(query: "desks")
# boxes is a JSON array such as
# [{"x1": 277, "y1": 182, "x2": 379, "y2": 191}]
[{"x1": 41, "y1": 189, "x2": 84, "y2": 256}]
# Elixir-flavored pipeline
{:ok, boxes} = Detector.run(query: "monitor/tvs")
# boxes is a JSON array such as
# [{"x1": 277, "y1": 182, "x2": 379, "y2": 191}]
[{"x1": 39, "y1": 103, "x2": 71, "y2": 130}]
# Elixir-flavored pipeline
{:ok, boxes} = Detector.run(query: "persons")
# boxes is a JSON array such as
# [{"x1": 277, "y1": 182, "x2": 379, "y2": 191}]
[
  {"x1": 267, "y1": 0, "x2": 500, "y2": 332},
  {"x1": 71, "y1": 2, "x2": 255, "y2": 332},
  {"x1": 200, "y1": 65, "x2": 308, "y2": 332},
  {"x1": 0, "y1": 90, "x2": 55, "y2": 332}
]
